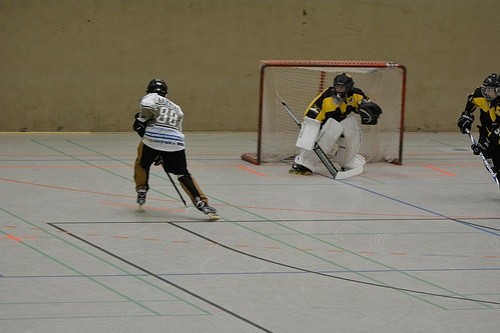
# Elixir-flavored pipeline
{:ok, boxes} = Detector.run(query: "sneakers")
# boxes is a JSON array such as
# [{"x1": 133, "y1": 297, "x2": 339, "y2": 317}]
[
  {"x1": 341, "y1": 166, "x2": 347, "y2": 171},
  {"x1": 289, "y1": 162, "x2": 313, "y2": 175},
  {"x1": 135, "y1": 184, "x2": 149, "y2": 211},
  {"x1": 194, "y1": 197, "x2": 220, "y2": 219}
]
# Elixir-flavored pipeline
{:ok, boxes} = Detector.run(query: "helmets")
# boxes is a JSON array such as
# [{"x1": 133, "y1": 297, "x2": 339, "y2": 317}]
[
  {"x1": 333, "y1": 72, "x2": 355, "y2": 97},
  {"x1": 146, "y1": 78, "x2": 168, "y2": 97},
  {"x1": 481, "y1": 73, "x2": 500, "y2": 103}
]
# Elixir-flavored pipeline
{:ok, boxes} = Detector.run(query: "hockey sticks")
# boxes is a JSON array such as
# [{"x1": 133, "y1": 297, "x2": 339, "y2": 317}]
[
  {"x1": 161, "y1": 162, "x2": 193, "y2": 208},
  {"x1": 282, "y1": 101, "x2": 363, "y2": 180},
  {"x1": 466, "y1": 127, "x2": 500, "y2": 187}
]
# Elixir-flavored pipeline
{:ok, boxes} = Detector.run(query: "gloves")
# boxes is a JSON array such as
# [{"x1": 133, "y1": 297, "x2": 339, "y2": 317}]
[
  {"x1": 133, "y1": 113, "x2": 150, "y2": 137},
  {"x1": 457, "y1": 111, "x2": 475, "y2": 134},
  {"x1": 471, "y1": 138, "x2": 491, "y2": 155}
]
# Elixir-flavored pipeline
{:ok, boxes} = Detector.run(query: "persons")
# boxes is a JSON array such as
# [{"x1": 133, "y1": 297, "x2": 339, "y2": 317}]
[
  {"x1": 133, "y1": 79, "x2": 220, "y2": 219},
  {"x1": 457, "y1": 73, "x2": 500, "y2": 188},
  {"x1": 289, "y1": 72, "x2": 383, "y2": 175}
]
